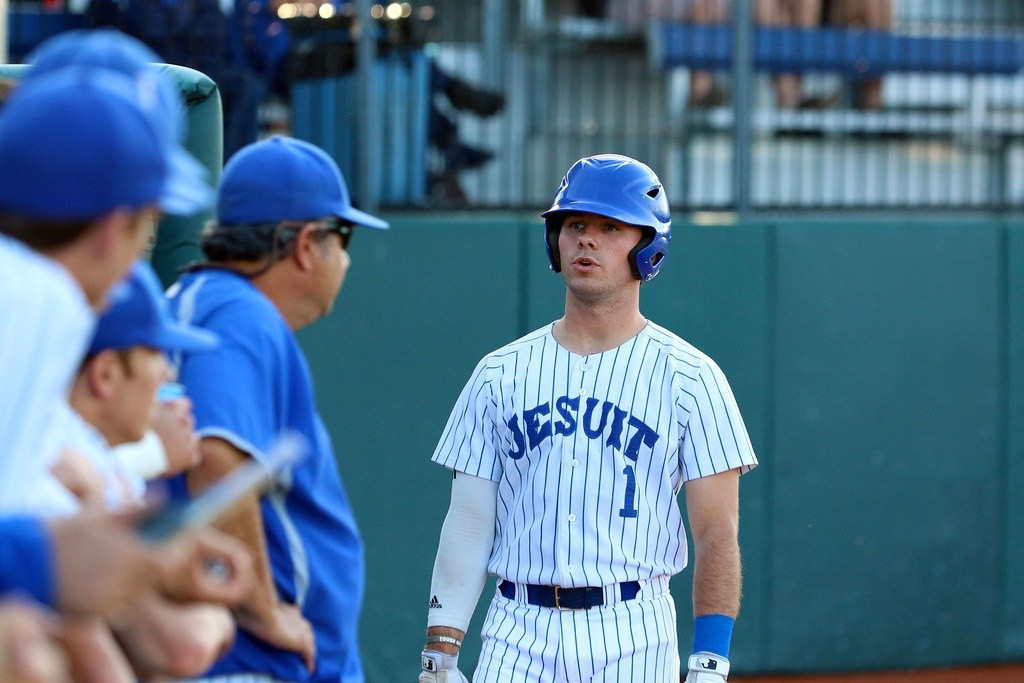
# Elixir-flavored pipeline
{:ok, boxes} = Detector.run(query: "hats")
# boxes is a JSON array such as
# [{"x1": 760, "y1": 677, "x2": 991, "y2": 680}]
[
  {"x1": 88, "y1": 262, "x2": 220, "y2": 357},
  {"x1": 215, "y1": 135, "x2": 391, "y2": 233},
  {"x1": 0, "y1": 29, "x2": 224, "y2": 218}
]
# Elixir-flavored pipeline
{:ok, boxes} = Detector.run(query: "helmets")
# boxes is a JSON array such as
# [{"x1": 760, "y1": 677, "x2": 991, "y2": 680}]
[{"x1": 542, "y1": 154, "x2": 673, "y2": 283}]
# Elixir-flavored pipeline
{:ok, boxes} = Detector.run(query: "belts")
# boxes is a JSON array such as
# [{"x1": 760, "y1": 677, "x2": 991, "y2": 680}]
[{"x1": 500, "y1": 581, "x2": 642, "y2": 609}]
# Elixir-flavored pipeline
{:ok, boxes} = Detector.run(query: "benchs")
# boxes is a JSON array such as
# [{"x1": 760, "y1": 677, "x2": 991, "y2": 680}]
[{"x1": 643, "y1": 17, "x2": 1023, "y2": 210}]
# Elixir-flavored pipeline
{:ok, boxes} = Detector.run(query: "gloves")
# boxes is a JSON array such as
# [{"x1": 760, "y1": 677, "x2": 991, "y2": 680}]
[
  {"x1": 683, "y1": 652, "x2": 731, "y2": 683},
  {"x1": 419, "y1": 649, "x2": 469, "y2": 683}
]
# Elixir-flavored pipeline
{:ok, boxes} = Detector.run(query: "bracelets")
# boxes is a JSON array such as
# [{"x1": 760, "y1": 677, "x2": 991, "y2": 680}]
[{"x1": 425, "y1": 636, "x2": 463, "y2": 648}]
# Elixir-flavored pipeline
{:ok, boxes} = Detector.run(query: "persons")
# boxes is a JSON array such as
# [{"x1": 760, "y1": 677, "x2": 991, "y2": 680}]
[
  {"x1": 5, "y1": 0, "x2": 506, "y2": 205},
  {"x1": 169, "y1": 134, "x2": 396, "y2": 683},
  {"x1": 420, "y1": 154, "x2": 759, "y2": 683},
  {"x1": 694, "y1": 2, "x2": 891, "y2": 109},
  {"x1": 0, "y1": 30, "x2": 255, "y2": 683}
]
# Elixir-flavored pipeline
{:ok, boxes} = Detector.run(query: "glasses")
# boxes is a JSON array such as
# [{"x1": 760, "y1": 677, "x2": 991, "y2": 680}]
[{"x1": 311, "y1": 220, "x2": 354, "y2": 249}]
